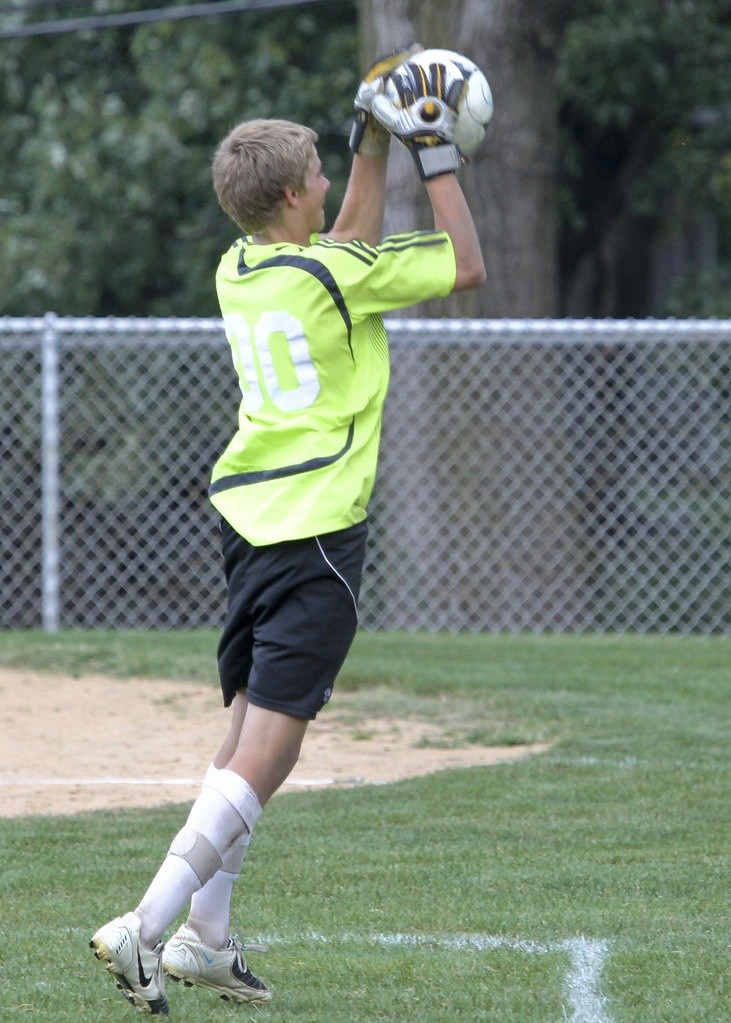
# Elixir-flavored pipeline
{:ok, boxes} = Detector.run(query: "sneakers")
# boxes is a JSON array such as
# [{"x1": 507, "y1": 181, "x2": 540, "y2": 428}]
[
  {"x1": 160, "y1": 923, "x2": 272, "y2": 1005},
  {"x1": 89, "y1": 912, "x2": 169, "y2": 1018}
]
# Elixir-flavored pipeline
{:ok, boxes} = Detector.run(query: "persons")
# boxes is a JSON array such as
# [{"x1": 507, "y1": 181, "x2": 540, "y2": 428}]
[{"x1": 87, "y1": 40, "x2": 487, "y2": 1014}]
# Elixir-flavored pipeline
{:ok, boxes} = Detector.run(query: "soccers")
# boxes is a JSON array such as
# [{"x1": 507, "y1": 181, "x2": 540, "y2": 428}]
[{"x1": 381, "y1": 47, "x2": 496, "y2": 170}]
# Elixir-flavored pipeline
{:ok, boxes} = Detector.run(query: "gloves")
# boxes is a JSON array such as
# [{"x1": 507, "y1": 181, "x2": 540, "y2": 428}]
[
  {"x1": 371, "y1": 60, "x2": 469, "y2": 181},
  {"x1": 349, "y1": 42, "x2": 426, "y2": 156}
]
